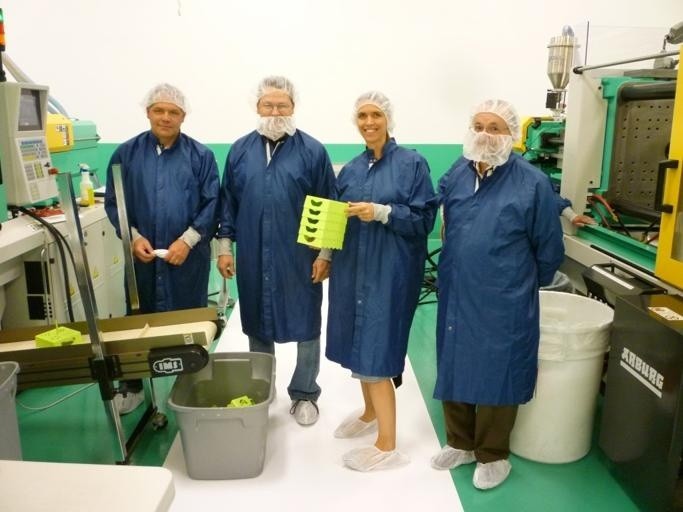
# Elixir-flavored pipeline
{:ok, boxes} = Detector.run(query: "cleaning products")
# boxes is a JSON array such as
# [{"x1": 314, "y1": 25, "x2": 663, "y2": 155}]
[{"x1": 78, "y1": 162, "x2": 95, "y2": 206}]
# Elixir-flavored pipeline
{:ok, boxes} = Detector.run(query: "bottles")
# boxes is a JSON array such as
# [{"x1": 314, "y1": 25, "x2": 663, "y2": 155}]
[{"x1": 77, "y1": 168, "x2": 95, "y2": 211}]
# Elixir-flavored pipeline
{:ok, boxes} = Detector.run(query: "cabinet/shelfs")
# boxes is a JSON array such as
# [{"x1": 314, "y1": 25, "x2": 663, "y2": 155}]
[{"x1": 0, "y1": 217, "x2": 127, "y2": 330}]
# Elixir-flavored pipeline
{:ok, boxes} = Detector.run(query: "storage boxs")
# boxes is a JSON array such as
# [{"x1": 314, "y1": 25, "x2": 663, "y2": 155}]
[{"x1": 167, "y1": 352, "x2": 276, "y2": 480}]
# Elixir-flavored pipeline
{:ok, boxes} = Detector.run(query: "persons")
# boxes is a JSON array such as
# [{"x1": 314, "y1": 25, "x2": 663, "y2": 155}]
[
  {"x1": 214, "y1": 75, "x2": 336, "y2": 426},
  {"x1": 325, "y1": 91, "x2": 439, "y2": 471},
  {"x1": 104, "y1": 82, "x2": 221, "y2": 415}
]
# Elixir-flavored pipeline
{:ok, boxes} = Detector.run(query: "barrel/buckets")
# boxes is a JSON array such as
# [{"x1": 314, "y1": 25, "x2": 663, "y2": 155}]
[{"x1": 510, "y1": 289, "x2": 615, "y2": 465}]
